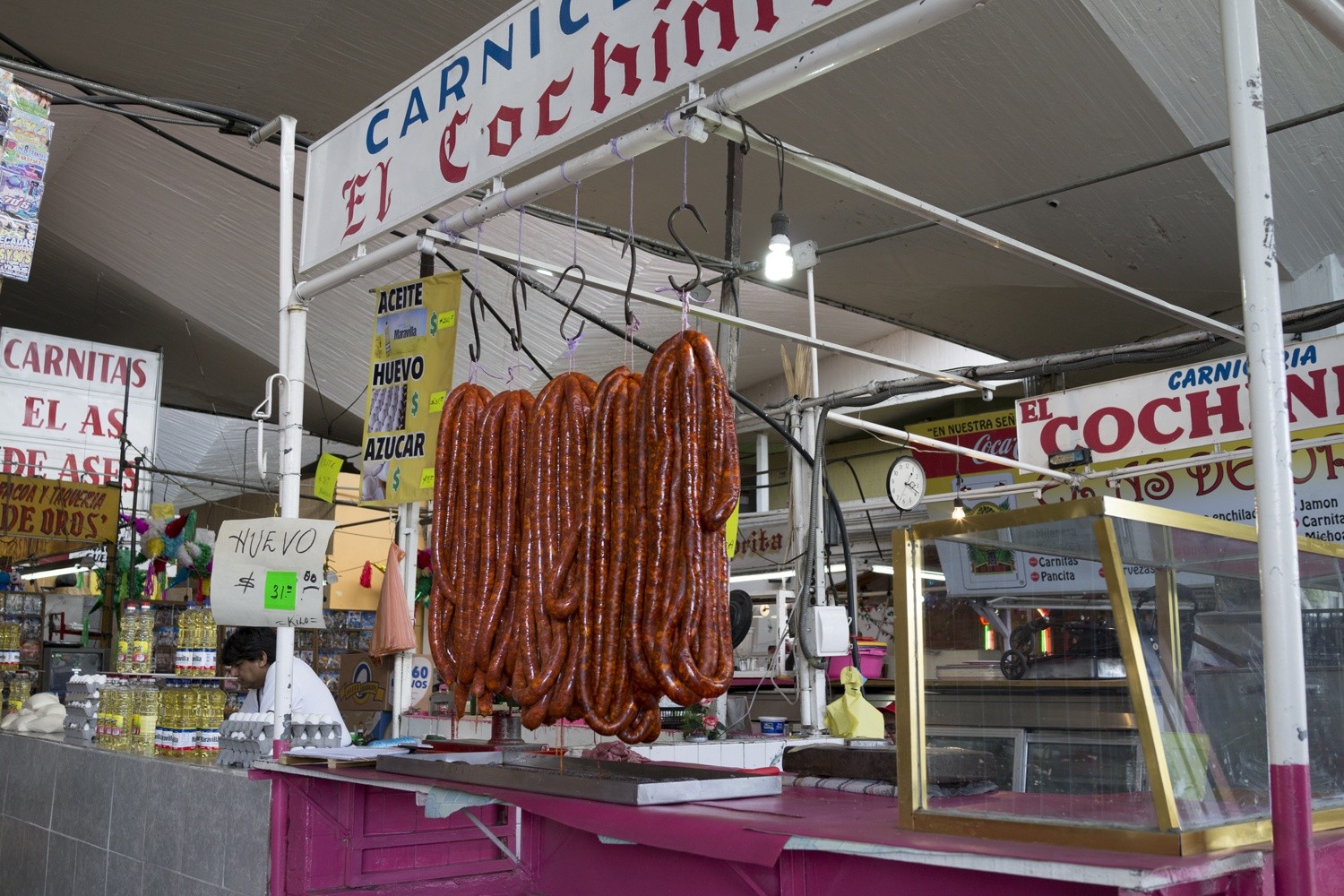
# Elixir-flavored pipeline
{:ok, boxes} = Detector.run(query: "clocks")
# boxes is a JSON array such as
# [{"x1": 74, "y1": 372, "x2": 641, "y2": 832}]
[{"x1": 886, "y1": 454, "x2": 928, "y2": 513}]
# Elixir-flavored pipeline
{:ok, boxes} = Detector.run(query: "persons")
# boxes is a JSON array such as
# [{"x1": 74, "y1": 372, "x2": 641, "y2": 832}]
[{"x1": 218, "y1": 624, "x2": 358, "y2": 750}]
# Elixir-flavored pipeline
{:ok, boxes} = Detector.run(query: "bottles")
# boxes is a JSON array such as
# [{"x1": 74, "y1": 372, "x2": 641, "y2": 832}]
[
  {"x1": 7, "y1": 669, "x2": 31, "y2": 716},
  {"x1": 174, "y1": 602, "x2": 217, "y2": 678},
  {"x1": 95, "y1": 675, "x2": 225, "y2": 760},
  {"x1": 351, "y1": 728, "x2": 366, "y2": 746},
  {"x1": 0, "y1": 615, "x2": 19, "y2": 716},
  {"x1": 115, "y1": 600, "x2": 153, "y2": 674}
]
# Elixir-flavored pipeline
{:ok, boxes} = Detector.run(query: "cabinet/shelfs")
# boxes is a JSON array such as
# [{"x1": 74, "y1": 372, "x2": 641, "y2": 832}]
[
  {"x1": 123, "y1": 599, "x2": 378, "y2": 717},
  {"x1": 1, "y1": 592, "x2": 46, "y2": 719}
]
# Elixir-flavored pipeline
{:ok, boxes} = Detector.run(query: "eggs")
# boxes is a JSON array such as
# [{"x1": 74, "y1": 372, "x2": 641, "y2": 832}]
[{"x1": 222, "y1": 711, "x2": 341, "y2": 764}]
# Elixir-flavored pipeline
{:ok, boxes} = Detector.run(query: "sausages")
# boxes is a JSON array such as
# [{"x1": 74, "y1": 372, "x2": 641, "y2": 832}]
[{"x1": 427, "y1": 334, "x2": 743, "y2": 745}]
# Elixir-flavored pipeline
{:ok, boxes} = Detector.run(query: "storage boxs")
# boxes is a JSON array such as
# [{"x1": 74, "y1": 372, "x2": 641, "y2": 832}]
[
  {"x1": 176, "y1": 471, "x2": 398, "y2": 610},
  {"x1": 337, "y1": 650, "x2": 434, "y2": 713},
  {"x1": 338, "y1": 708, "x2": 393, "y2": 741}
]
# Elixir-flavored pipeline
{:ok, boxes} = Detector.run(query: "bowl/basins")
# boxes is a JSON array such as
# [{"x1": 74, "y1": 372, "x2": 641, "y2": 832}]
[{"x1": 758, "y1": 715, "x2": 787, "y2": 736}]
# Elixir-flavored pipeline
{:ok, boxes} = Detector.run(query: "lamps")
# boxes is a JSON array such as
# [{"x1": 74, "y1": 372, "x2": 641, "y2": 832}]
[
  {"x1": 764, "y1": 141, "x2": 796, "y2": 283},
  {"x1": 951, "y1": 452, "x2": 967, "y2": 522}
]
